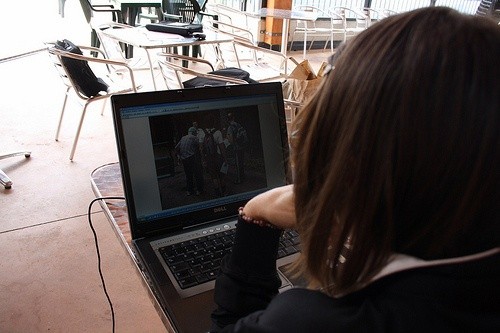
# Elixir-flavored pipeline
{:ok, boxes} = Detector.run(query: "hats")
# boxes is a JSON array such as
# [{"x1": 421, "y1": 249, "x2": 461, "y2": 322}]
[
  {"x1": 188, "y1": 127, "x2": 196, "y2": 134},
  {"x1": 227, "y1": 113, "x2": 234, "y2": 119}
]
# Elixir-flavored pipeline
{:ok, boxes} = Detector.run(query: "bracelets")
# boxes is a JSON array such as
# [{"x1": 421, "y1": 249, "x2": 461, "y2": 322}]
[{"x1": 238, "y1": 207, "x2": 282, "y2": 230}]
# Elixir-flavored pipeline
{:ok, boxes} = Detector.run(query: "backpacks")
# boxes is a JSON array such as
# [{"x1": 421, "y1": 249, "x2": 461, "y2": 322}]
[
  {"x1": 202, "y1": 128, "x2": 218, "y2": 162},
  {"x1": 230, "y1": 122, "x2": 248, "y2": 147}
]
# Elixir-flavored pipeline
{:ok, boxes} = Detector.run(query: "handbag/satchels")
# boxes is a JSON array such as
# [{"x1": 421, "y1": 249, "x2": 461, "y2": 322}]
[
  {"x1": 280, "y1": 56, "x2": 331, "y2": 108},
  {"x1": 145, "y1": 22, "x2": 203, "y2": 37}
]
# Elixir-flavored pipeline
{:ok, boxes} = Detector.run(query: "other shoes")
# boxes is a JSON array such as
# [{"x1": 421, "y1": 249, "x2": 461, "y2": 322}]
[
  {"x1": 198, "y1": 190, "x2": 207, "y2": 194},
  {"x1": 185, "y1": 191, "x2": 192, "y2": 196}
]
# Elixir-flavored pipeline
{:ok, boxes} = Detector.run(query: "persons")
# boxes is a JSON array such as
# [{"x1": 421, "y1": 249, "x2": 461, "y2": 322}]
[
  {"x1": 209, "y1": 8, "x2": 500, "y2": 333},
  {"x1": 173, "y1": 113, "x2": 230, "y2": 198}
]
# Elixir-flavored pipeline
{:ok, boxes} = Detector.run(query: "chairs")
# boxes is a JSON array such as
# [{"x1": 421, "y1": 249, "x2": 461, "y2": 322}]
[{"x1": 44, "y1": 0, "x2": 399, "y2": 162}]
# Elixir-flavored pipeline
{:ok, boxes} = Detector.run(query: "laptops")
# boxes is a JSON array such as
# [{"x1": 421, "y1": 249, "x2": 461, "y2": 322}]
[{"x1": 110, "y1": 82, "x2": 311, "y2": 333}]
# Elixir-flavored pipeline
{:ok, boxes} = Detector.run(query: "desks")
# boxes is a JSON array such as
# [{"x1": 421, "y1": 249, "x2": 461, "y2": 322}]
[
  {"x1": 250, "y1": 8, "x2": 320, "y2": 55},
  {"x1": 114, "y1": 0, "x2": 186, "y2": 59},
  {"x1": 104, "y1": 27, "x2": 234, "y2": 91},
  {"x1": 90, "y1": 162, "x2": 214, "y2": 333}
]
